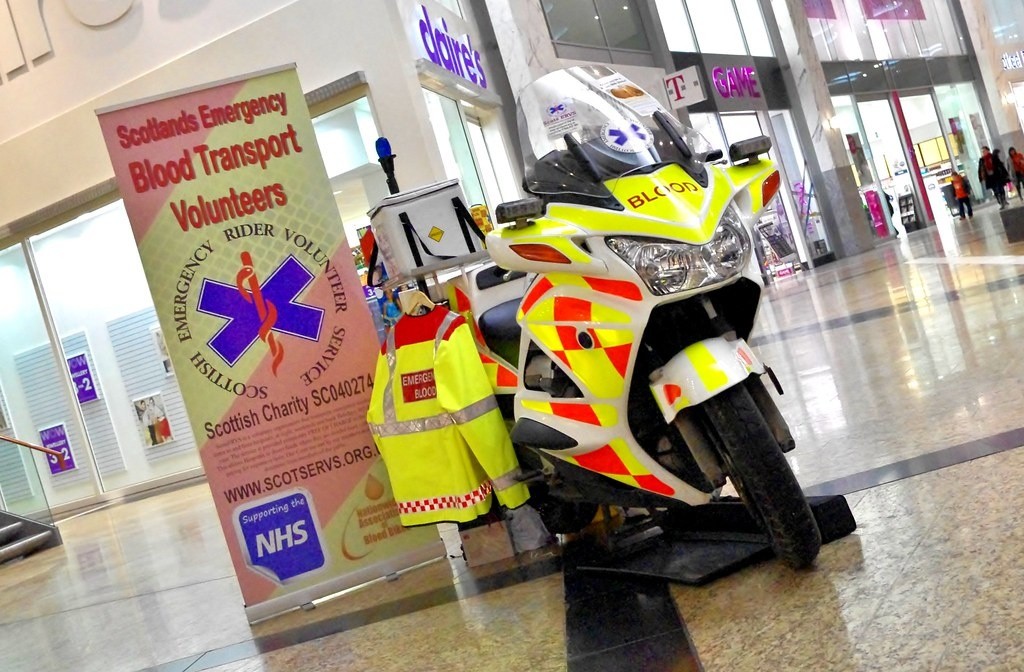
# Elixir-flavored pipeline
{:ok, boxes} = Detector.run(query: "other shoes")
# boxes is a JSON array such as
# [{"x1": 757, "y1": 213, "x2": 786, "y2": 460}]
[
  {"x1": 959, "y1": 217, "x2": 965, "y2": 220},
  {"x1": 969, "y1": 216, "x2": 973, "y2": 219},
  {"x1": 999, "y1": 206, "x2": 1004, "y2": 210}
]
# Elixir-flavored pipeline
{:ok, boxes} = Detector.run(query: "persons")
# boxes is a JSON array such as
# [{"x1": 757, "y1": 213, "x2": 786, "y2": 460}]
[
  {"x1": 951, "y1": 171, "x2": 973, "y2": 220},
  {"x1": 883, "y1": 191, "x2": 899, "y2": 235},
  {"x1": 978, "y1": 146, "x2": 1024, "y2": 210},
  {"x1": 382, "y1": 283, "x2": 423, "y2": 327},
  {"x1": 141, "y1": 398, "x2": 164, "y2": 445}
]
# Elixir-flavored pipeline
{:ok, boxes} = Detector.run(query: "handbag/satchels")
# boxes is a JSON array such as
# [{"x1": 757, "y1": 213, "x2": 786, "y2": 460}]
[{"x1": 1005, "y1": 182, "x2": 1019, "y2": 198}]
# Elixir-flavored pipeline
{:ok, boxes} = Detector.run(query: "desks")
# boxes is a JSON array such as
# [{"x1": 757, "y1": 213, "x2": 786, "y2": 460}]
[{"x1": 381, "y1": 249, "x2": 489, "y2": 300}]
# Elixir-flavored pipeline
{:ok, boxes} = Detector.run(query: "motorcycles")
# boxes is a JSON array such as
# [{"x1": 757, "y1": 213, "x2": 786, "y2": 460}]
[{"x1": 447, "y1": 61, "x2": 825, "y2": 578}]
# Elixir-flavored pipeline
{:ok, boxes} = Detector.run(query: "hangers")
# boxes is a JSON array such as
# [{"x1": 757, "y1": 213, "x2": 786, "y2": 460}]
[{"x1": 405, "y1": 279, "x2": 435, "y2": 315}]
